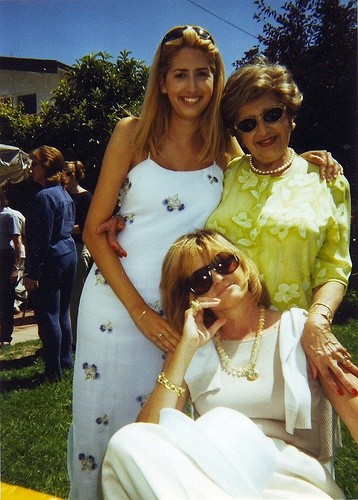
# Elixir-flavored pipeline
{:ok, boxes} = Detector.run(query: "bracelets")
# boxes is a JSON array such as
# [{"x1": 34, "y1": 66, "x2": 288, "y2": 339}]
[
  {"x1": 308, "y1": 303, "x2": 335, "y2": 316},
  {"x1": 133, "y1": 304, "x2": 150, "y2": 323},
  {"x1": 157, "y1": 372, "x2": 186, "y2": 398}
]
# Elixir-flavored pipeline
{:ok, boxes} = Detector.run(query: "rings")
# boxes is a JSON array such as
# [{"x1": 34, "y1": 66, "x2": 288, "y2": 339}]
[{"x1": 158, "y1": 333, "x2": 163, "y2": 339}]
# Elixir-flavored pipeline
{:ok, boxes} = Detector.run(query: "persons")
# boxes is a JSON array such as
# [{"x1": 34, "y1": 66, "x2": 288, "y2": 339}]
[
  {"x1": 100, "y1": 227, "x2": 358, "y2": 500},
  {"x1": 22, "y1": 145, "x2": 78, "y2": 382},
  {"x1": 58, "y1": 159, "x2": 94, "y2": 354},
  {"x1": 0, "y1": 187, "x2": 29, "y2": 346},
  {"x1": 98, "y1": 62, "x2": 358, "y2": 399},
  {"x1": 66, "y1": 24, "x2": 344, "y2": 500}
]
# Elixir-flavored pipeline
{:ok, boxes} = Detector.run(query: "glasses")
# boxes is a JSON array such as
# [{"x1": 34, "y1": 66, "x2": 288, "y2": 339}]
[
  {"x1": 180, "y1": 253, "x2": 239, "y2": 296},
  {"x1": 161, "y1": 26, "x2": 214, "y2": 54},
  {"x1": 31, "y1": 161, "x2": 40, "y2": 168},
  {"x1": 234, "y1": 102, "x2": 287, "y2": 133}
]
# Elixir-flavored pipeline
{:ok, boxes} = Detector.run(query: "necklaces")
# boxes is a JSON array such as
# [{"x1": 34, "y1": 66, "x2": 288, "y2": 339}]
[
  {"x1": 249, "y1": 145, "x2": 296, "y2": 173},
  {"x1": 214, "y1": 305, "x2": 267, "y2": 381}
]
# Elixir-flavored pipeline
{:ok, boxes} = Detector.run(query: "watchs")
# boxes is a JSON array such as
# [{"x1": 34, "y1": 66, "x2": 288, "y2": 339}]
[{"x1": 309, "y1": 308, "x2": 333, "y2": 325}]
